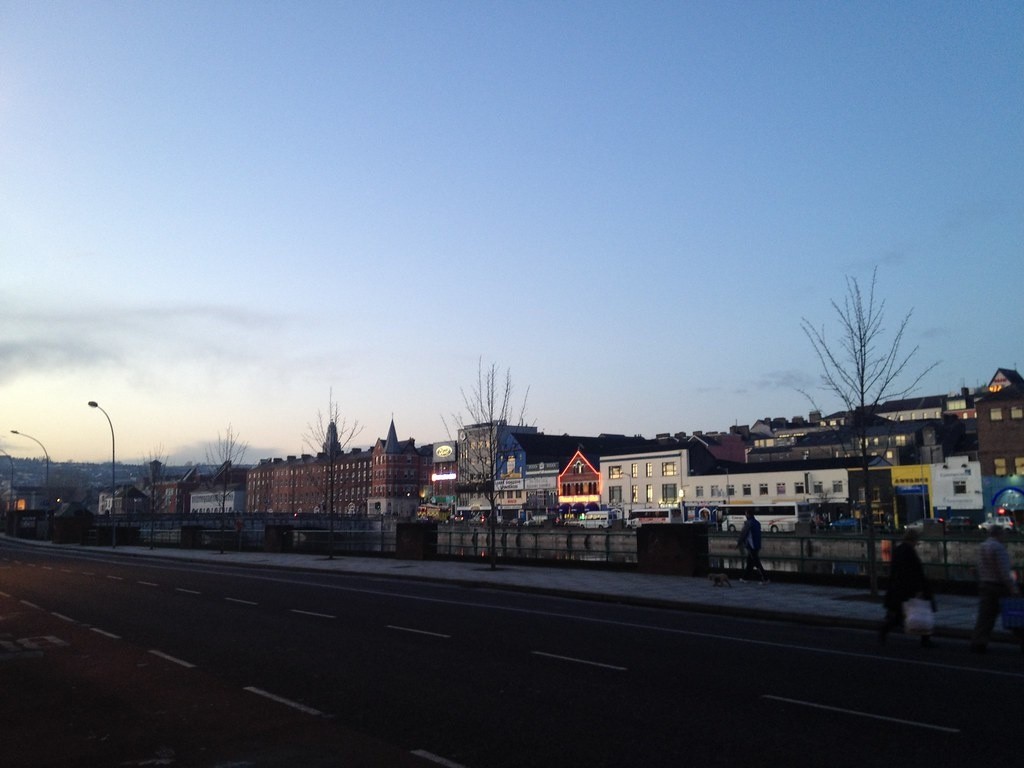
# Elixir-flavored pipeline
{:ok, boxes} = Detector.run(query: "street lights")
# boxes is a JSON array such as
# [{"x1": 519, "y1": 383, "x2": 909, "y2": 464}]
[
  {"x1": 88, "y1": 401, "x2": 117, "y2": 550},
  {"x1": 12, "y1": 429, "x2": 52, "y2": 538}
]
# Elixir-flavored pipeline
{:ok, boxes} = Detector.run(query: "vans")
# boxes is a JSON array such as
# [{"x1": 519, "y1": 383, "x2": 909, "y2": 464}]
[{"x1": 579, "y1": 509, "x2": 617, "y2": 529}]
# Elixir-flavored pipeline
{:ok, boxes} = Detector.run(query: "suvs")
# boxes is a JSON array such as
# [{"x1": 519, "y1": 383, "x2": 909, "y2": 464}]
[
  {"x1": 947, "y1": 516, "x2": 973, "y2": 534},
  {"x1": 977, "y1": 516, "x2": 1021, "y2": 535}
]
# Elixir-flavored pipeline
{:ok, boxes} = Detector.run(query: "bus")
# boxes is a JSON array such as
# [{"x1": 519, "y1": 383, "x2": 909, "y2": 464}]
[
  {"x1": 713, "y1": 503, "x2": 811, "y2": 534},
  {"x1": 626, "y1": 508, "x2": 682, "y2": 531}
]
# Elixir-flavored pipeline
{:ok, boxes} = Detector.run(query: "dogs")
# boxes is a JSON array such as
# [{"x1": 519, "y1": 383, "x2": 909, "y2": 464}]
[{"x1": 707, "y1": 573, "x2": 732, "y2": 588}]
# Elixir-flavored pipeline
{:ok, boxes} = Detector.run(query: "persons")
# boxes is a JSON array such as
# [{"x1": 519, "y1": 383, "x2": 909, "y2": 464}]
[
  {"x1": 727, "y1": 508, "x2": 774, "y2": 586},
  {"x1": 808, "y1": 513, "x2": 835, "y2": 536},
  {"x1": 872, "y1": 528, "x2": 941, "y2": 647},
  {"x1": 964, "y1": 525, "x2": 1024, "y2": 658}
]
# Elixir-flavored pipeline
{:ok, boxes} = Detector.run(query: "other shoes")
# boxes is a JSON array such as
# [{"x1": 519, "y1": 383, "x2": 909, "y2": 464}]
[
  {"x1": 739, "y1": 579, "x2": 752, "y2": 585},
  {"x1": 757, "y1": 580, "x2": 770, "y2": 586}
]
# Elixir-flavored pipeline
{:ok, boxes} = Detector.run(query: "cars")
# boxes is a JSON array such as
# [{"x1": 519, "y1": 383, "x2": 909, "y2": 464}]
[
  {"x1": 905, "y1": 516, "x2": 947, "y2": 533},
  {"x1": 405, "y1": 515, "x2": 575, "y2": 527},
  {"x1": 829, "y1": 518, "x2": 869, "y2": 528}
]
